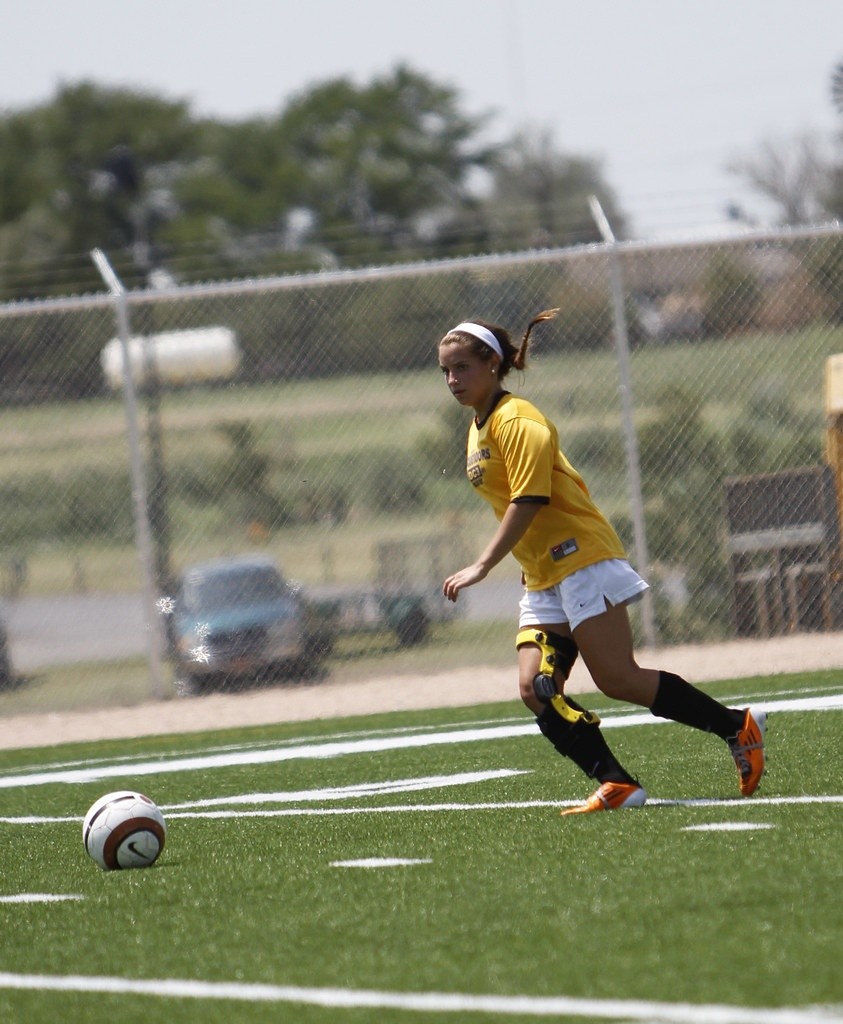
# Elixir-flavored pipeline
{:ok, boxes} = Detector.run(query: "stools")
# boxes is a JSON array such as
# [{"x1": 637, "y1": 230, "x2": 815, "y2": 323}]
[
  {"x1": 738, "y1": 569, "x2": 775, "y2": 637},
  {"x1": 781, "y1": 561, "x2": 825, "y2": 635}
]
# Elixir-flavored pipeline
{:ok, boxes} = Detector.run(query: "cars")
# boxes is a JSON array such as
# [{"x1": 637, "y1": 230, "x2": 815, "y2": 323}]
[{"x1": 173, "y1": 558, "x2": 316, "y2": 692}]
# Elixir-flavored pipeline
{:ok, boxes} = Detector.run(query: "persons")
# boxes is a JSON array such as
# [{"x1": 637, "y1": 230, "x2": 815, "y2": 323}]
[{"x1": 438, "y1": 312, "x2": 767, "y2": 816}]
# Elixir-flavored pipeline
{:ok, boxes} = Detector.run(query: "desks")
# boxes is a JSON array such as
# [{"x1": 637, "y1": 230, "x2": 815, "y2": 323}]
[{"x1": 724, "y1": 523, "x2": 826, "y2": 638}]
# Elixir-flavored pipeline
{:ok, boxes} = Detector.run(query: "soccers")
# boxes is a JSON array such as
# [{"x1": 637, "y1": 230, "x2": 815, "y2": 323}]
[{"x1": 81, "y1": 789, "x2": 167, "y2": 872}]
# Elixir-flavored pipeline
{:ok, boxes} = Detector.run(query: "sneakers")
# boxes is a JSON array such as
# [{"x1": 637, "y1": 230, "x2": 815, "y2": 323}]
[
  {"x1": 725, "y1": 707, "x2": 769, "y2": 798},
  {"x1": 559, "y1": 781, "x2": 649, "y2": 817}
]
